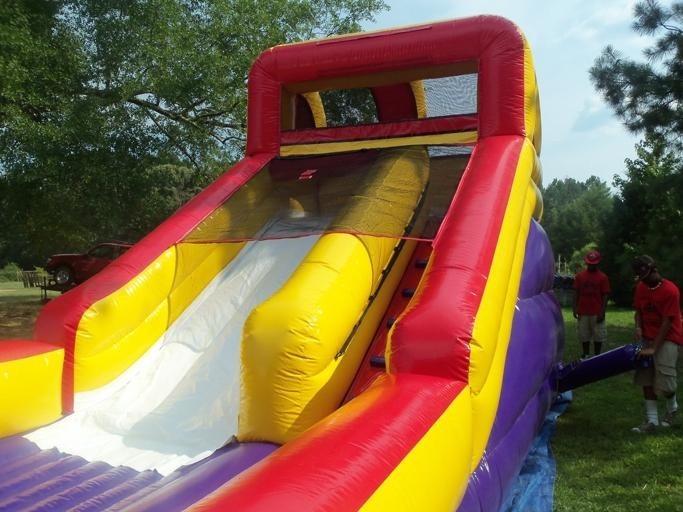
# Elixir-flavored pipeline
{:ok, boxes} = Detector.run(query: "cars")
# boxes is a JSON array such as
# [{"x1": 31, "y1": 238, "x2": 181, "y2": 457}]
[{"x1": 47, "y1": 243, "x2": 132, "y2": 285}]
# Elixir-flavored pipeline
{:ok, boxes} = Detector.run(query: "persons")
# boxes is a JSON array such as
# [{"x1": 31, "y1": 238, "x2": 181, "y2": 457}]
[
  {"x1": 630, "y1": 253, "x2": 682, "y2": 433},
  {"x1": 571, "y1": 250, "x2": 610, "y2": 360}
]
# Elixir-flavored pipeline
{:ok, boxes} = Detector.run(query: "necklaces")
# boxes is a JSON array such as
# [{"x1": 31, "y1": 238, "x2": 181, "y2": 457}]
[{"x1": 647, "y1": 278, "x2": 664, "y2": 291}]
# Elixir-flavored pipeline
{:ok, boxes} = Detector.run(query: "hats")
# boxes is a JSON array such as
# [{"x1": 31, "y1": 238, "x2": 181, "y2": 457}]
[
  {"x1": 632, "y1": 255, "x2": 654, "y2": 282},
  {"x1": 585, "y1": 251, "x2": 601, "y2": 265}
]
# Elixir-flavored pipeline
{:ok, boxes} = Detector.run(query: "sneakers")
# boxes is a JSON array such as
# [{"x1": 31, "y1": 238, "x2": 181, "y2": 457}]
[
  {"x1": 630, "y1": 423, "x2": 658, "y2": 433},
  {"x1": 661, "y1": 412, "x2": 673, "y2": 427}
]
